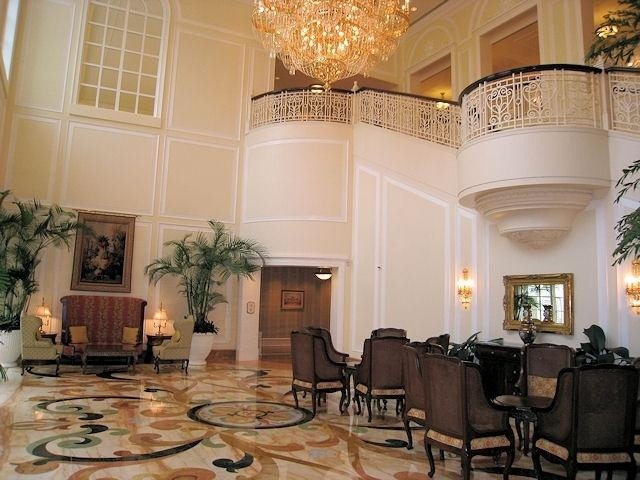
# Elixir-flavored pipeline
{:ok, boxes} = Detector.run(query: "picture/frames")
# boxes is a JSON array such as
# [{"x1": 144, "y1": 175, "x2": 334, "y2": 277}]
[
  {"x1": 281, "y1": 290, "x2": 304, "y2": 310},
  {"x1": 70, "y1": 212, "x2": 135, "y2": 292}
]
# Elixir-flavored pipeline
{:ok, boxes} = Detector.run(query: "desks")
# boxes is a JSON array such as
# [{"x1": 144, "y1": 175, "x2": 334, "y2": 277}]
[
  {"x1": 40, "y1": 332, "x2": 55, "y2": 344},
  {"x1": 147, "y1": 334, "x2": 169, "y2": 364}
]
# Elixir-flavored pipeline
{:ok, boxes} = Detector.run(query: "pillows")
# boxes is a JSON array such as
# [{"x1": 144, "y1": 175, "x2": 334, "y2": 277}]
[
  {"x1": 122, "y1": 325, "x2": 138, "y2": 343},
  {"x1": 70, "y1": 326, "x2": 88, "y2": 344}
]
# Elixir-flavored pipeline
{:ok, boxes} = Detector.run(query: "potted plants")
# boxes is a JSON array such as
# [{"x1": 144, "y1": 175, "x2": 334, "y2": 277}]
[
  {"x1": 0, "y1": 189, "x2": 97, "y2": 369},
  {"x1": 142, "y1": 219, "x2": 270, "y2": 365}
]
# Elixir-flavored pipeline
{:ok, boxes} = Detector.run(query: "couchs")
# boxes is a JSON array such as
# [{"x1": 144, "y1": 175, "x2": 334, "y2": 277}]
[{"x1": 61, "y1": 296, "x2": 148, "y2": 365}]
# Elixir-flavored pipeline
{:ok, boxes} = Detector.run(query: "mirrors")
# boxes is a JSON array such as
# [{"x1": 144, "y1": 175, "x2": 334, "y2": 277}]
[{"x1": 503, "y1": 273, "x2": 573, "y2": 334}]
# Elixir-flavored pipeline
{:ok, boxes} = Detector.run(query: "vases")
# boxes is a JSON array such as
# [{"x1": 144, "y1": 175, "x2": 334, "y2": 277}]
[
  {"x1": 519, "y1": 305, "x2": 536, "y2": 345},
  {"x1": 542, "y1": 303, "x2": 553, "y2": 322}
]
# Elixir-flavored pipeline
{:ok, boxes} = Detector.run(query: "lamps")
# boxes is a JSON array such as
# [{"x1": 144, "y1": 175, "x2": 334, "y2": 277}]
[
  {"x1": 35, "y1": 297, "x2": 52, "y2": 332},
  {"x1": 625, "y1": 257, "x2": 640, "y2": 316},
  {"x1": 251, "y1": 0, "x2": 411, "y2": 92},
  {"x1": 457, "y1": 268, "x2": 473, "y2": 309},
  {"x1": 309, "y1": 84, "x2": 324, "y2": 94},
  {"x1": 153, "y1": 303, "x2": 167, "y2": 335},
  {"x1": 437, "y1": 93, "x2": 449, "y2": 109},
  {"x1": 313, "y1": 269, "x2": 332, "y2": 280},
  {"x1": 595, "y1": 25, "x2": 620, "y2": 39}
]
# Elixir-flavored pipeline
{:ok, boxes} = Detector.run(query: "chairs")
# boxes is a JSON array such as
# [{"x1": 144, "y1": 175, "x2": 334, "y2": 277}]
[
  {"x1": 152, "y1": 316, "x2": 195, "y2": 374},
  {"x1": 21, "y1": 296, "x2": 59, "y2": 377}
]
